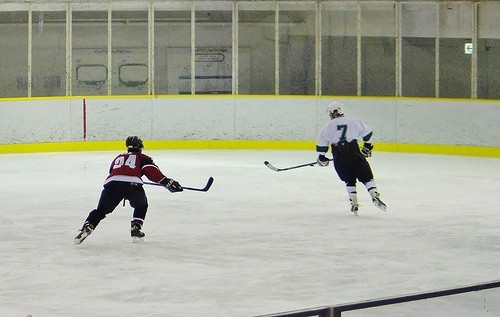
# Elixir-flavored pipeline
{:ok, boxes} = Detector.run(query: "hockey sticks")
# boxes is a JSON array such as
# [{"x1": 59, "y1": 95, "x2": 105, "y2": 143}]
[
  {"x1": 143, "y1": 177, "x2": 214, "y2": 192},
  {"x1": 264, "y1": 159, "x2": 333, "y2": 172}
]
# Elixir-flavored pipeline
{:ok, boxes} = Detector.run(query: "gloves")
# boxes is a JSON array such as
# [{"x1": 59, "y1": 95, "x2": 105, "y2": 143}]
[
  {"x1": 360, "y1": 144, "x2": 373, "y2": 158},
  {"x1": 317, "y1": 155, "x2": 329, "y2": 167},
  {"x1": 161, "y1": 178, "x2": 183, "y2": 193}
]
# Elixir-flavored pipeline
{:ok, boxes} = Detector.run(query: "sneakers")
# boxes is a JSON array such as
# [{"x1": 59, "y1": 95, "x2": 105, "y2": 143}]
[
  {"x1": 349, "y1": 196, "x2": 359, "y2": 217},
  {"x1": 367, "y1": 187, "x2": 387, "y2": 212},
  {"x1": 131, "y1": 221, "x2": 145, "y2": 243},
  {"x1": 72, "y1": 224, "x2": 94, "y2": 246}
]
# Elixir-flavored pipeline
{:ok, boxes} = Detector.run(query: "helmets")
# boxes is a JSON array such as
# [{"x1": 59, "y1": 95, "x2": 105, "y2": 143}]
[
  {"x1": 327, "y1": 101, "x2": 344, "y2": 115},
  {"x1": 126, "y1": 136, "x2": 144, "y2": 152}
]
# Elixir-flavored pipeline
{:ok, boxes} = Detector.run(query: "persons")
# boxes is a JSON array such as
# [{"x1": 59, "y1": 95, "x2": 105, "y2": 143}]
[
  {"x1": 72, "y1": 135, "x2": 183, "y2": 246},
  {"x1": 316, "y1": 102, "x2": 387, "y2": 216}
]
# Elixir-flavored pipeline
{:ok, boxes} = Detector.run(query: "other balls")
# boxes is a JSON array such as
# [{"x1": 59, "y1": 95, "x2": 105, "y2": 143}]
[{"x1": 310, "y1": 164, "x2": 314, "y2": 166}]
